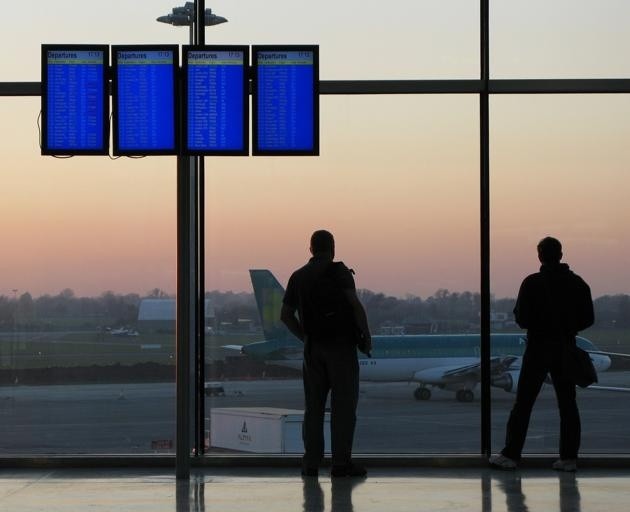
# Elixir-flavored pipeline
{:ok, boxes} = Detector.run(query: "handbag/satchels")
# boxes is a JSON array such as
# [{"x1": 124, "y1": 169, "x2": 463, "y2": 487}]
[{"x1": 573, "y1": 348, "x2": 599, "y2": 388}]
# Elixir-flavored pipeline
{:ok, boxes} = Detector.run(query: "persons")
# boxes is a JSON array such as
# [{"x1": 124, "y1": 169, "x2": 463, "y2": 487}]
[
  {"x1": 279, "y1": 229, "x2": 373, "y2": 479},
  {"x1": 488, "y1": 235, "x2": 595, "y2": 472}
]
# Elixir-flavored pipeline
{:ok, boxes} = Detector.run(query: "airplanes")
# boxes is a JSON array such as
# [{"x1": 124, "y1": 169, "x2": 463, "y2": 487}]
[{"x1": 241, "y1": 268, "x2": 630, "y2": 404}]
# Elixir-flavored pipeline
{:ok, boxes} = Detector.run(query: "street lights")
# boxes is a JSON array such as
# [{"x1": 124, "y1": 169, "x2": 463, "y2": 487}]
[{"x1": 158, "y1": 3, "x2": 222, "y2": 454}]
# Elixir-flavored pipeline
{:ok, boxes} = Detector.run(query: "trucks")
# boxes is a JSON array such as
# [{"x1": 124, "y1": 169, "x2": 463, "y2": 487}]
[{"x1": 193, "y1": 406, "x2": 330, "y2": 455}]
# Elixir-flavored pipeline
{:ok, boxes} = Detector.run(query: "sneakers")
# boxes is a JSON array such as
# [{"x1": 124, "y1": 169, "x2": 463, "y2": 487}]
[
  {"x1": 487, "y1": 454, "x2": 519, "y2": 470},
  {"x1": 330, "y1": 462, "x2": 369, "y2": 478},
  {"x1": 551, "y1": 458, "x2": 577, "y2": 473},
  {"x1": 301, "y1": 459, "x2": 319, "y2": 477}
]
